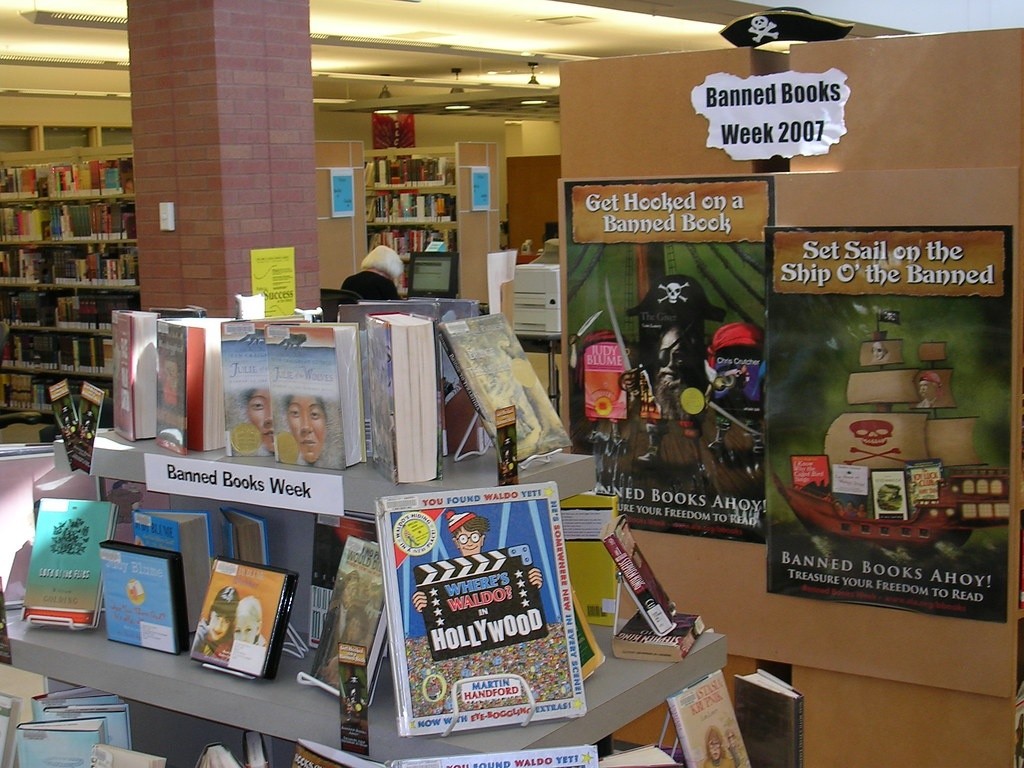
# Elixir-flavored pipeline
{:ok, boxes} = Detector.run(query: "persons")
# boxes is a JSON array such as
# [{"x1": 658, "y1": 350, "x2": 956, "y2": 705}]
[
  {"x1": 340, "y1": 245, "x2": 405, "y2": 300},
  {"x1": 281, "y1": 394, "x2": 327, "y2": 465},
  {"x1": 191, "y1": 586, "x2": 240, "y2": 664},
  {"x1": 241, "y1": 388, "x2": 275, "y2": 454},
  {"x1": 212, "y1": 595, "x2": 268, "y2": 662}
]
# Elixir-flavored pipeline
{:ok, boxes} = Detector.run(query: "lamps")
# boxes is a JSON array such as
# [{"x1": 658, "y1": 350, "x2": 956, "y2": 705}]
[
  {"x1": 520, "y1": 62, "x2": 548, "y2": 106},
  {"x1": 371, "y1": 74, "x2": 399, "y2": 115},
  {"x1": 444, "y1": 67, "x2": 471, "y2": 111}
]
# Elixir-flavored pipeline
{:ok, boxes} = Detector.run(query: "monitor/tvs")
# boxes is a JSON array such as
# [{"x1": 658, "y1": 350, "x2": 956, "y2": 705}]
[{"x1": 406, "y1": 251, "x2": 459, "y2": 300}]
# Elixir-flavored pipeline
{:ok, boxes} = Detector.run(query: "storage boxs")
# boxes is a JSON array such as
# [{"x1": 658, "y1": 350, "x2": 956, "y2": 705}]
[{"x1": 0, "y1": 157, "x2": 138, "y2": 413}]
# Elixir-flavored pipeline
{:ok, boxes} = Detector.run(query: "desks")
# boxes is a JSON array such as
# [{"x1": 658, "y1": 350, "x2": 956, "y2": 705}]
[{"x1": 513, "y1": 330, "x2": 561, "y2": 420}]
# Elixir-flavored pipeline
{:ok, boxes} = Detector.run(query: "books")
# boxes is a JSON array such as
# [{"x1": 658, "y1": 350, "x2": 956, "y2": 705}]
[
  {"x1": 1, "y1": 296, "x2": 804, "y2": 768},
  {"x1": 364, "y1": 156, "x2": 456, "y2": 257},
  {"x1": 0, "y1": 157, "x2": 140, "y2": 412}
]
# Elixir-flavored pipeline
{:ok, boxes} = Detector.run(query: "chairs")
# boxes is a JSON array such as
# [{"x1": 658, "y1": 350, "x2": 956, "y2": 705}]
[{"x1": 320, "y1": 288, "x2": 363, "y2": 322}]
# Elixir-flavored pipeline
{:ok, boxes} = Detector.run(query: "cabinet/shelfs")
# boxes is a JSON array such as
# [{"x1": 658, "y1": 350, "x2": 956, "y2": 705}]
[
  {"x1": 365, "y1": 141, "x2": 500, "y2": 308},
  {"x1": 0, "y1": 140, "x2": 141, "y2": 417},
  {"x1": 0, "y1": 288, "x2": 728, "y2": 768}
]
